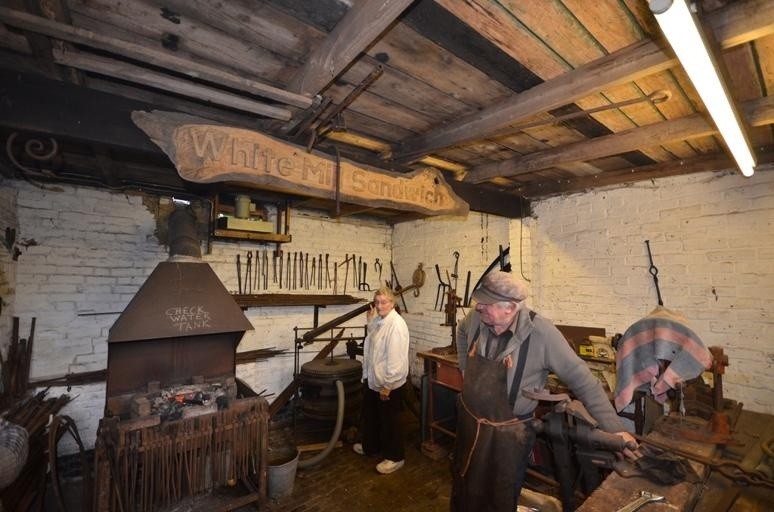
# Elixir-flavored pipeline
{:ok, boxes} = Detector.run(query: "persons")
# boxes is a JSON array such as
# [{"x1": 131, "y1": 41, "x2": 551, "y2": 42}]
[
  {"x1": 453, "y1": 270, "x2": 642, "y2": 512},
  {"x1": 353, "y1": 287, "x2": 410, "y2": 474}
]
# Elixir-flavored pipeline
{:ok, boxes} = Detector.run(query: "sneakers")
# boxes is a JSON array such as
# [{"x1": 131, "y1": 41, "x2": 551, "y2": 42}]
[
  {"x1": 375, "y1": 457, "x2": 404, "y2": 475},
  {"x1": 353, "y1": 442, "x2": 364, "y2": 454}
]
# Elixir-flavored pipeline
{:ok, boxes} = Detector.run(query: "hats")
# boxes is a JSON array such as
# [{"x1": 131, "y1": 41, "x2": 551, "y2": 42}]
[{"x1": 471, "y1": 270, "x2": 529, "y2": 305}]
